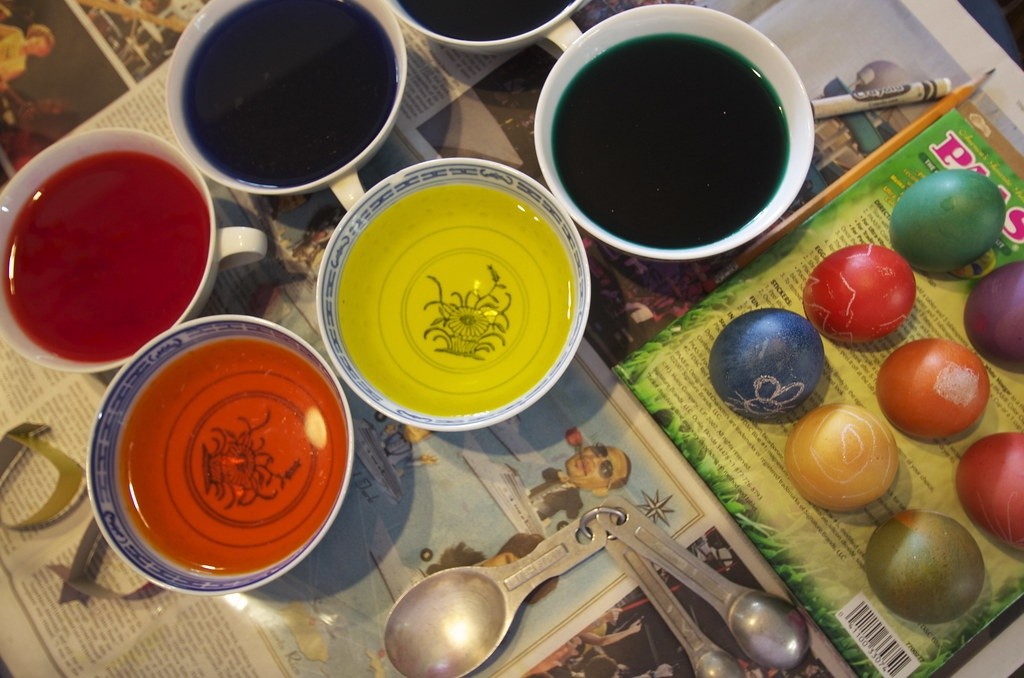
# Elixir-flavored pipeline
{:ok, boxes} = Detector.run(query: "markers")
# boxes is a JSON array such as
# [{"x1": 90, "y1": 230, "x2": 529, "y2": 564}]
[{"x1": 809, "y1": 78, "x2": 952, "y2": 120}]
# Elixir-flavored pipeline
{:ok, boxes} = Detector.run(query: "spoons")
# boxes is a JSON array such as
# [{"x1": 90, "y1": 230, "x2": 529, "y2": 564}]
[
  {"x1": 603, "y1": 535, "x2": 743, "y2": 678},
  {"x1": 601, "y1": 500, "x2": 809, "y2": 671},
  {"x1": 383, "y1": 516, "x2": 608, "y2": 678}
]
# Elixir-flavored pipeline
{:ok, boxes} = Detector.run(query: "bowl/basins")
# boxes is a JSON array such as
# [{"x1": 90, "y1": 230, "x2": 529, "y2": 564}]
[
  {"x1": 315, "y1": 157, "x2": 589, "y2": 432},
  {"x1": 87, "y1": 315, "x2": 354, "y2": 598},
  {"x1": 534, "y1": 4, "x2": 814, "y2": 261}
]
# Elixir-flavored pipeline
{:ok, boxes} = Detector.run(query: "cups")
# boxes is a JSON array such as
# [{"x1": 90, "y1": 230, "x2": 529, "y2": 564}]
[
  {"x1": 389, "y1": 0, "x2": 593, "y2": 59},
  {"x1": 1, "y1": 127, "x2": 267, "y2": 374},
  {"x1": 164, "y1": 0, "x2": 407, "y2": 211}
]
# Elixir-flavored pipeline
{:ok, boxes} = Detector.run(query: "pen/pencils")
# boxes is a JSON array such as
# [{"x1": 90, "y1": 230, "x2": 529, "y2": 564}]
[{"x1": 704, "y1": 66, "x2": 994, "y2": 292}]
